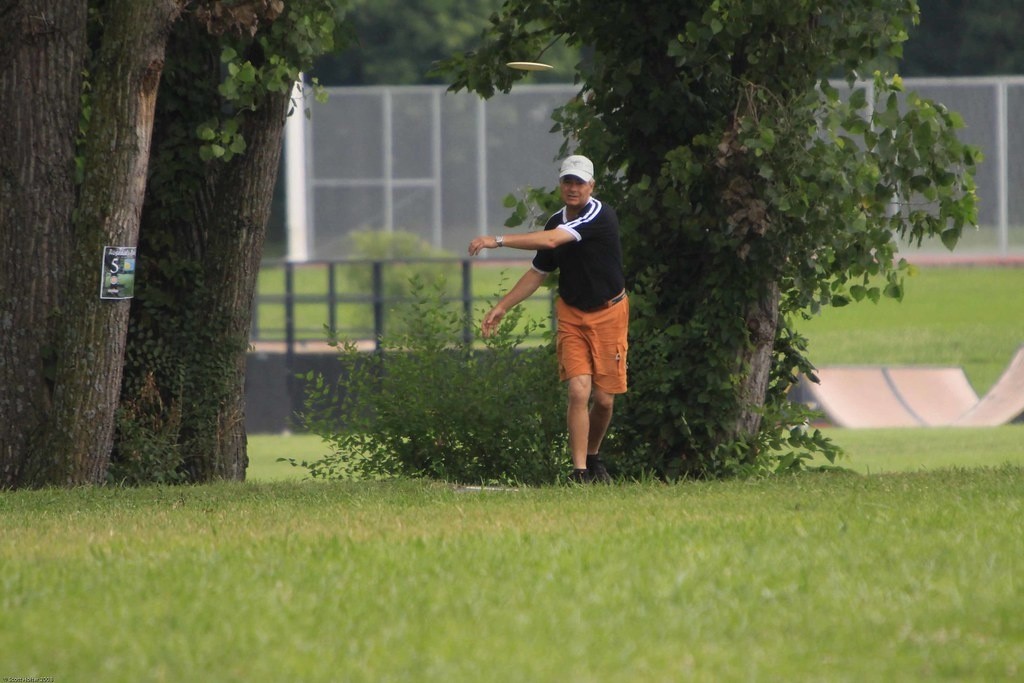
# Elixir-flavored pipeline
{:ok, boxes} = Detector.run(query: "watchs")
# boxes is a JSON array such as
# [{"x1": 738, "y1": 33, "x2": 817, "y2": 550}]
[{"x1": 496, "y1": 235, "x2": 503, "y2": 247}]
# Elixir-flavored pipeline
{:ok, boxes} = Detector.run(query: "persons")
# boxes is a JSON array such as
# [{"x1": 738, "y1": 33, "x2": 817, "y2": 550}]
[{"x1": 469, "y1": 156, "x2": 630, "y2": 481}]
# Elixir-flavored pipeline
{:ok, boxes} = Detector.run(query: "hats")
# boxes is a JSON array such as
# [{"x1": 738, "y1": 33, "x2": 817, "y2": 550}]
[{"x1": 559, "y1": 155, "x2": 594, "y2": 182}]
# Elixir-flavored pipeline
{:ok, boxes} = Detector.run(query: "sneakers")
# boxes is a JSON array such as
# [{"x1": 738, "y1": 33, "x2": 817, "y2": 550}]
[
  {"x1": 568, "y1": 472, "x2": 590, "y2": 484},
  {"x1": 588, "y1": 458, "x2": 613, "y2": 486}
]
